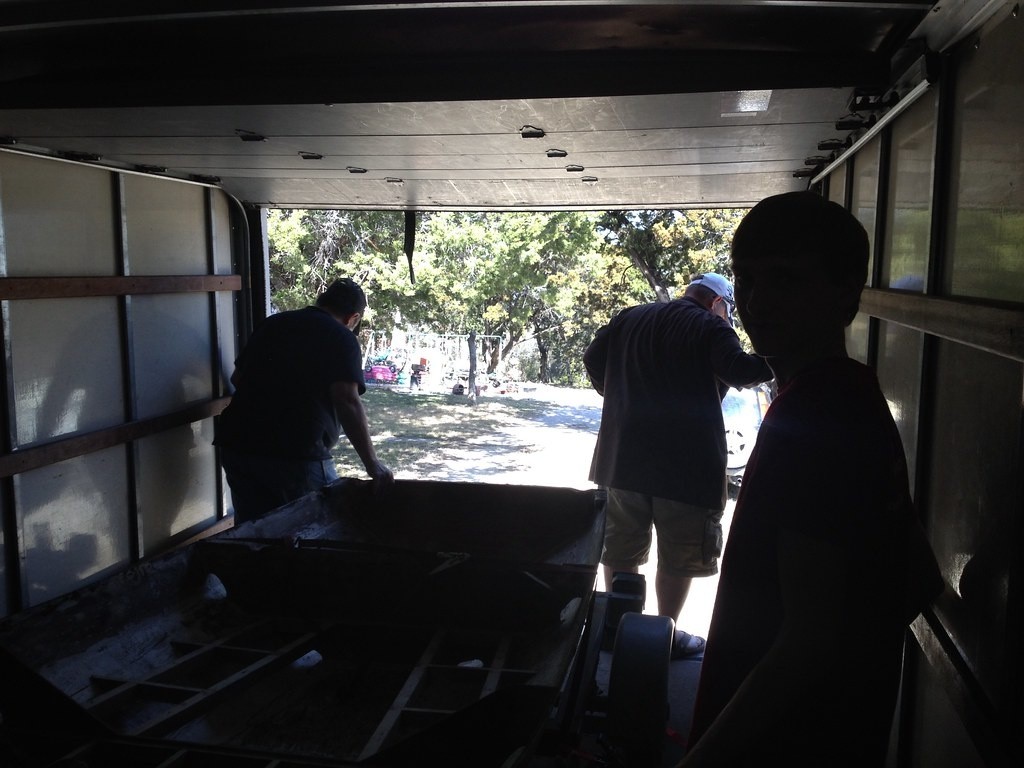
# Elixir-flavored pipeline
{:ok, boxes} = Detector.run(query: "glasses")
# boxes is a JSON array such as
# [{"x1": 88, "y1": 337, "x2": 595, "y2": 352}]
[{"x1": 737, "y1": 257, "x2": 830, "y2": 292}]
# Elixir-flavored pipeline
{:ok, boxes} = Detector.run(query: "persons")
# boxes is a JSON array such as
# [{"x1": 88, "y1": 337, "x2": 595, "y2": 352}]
[
  {"x1": 687, "y1": 191, "x2": 944, "y2": 768},
  {"x1": 213, "y1": 276, "x2": 396, "y2": 525},
  {"x1": 583, "y1": 273, "x2": 775, "y2": 660}
]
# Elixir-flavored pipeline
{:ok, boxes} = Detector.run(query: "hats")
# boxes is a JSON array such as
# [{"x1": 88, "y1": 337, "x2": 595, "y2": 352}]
[
  {"x1": 324, "y1": 278, "x2": 365, "y2": 336},
  {"x1": 689, "y1": 273, "x2": 736, "y2": 318}
]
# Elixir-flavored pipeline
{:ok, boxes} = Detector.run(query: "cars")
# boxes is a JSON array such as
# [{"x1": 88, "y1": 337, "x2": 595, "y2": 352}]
[
  {"x1": 363, "y1": 366, "x2": 399, "y2": 382},
  {"x1": 717, "y1": 384, "x2": 762, "y2": 489}
]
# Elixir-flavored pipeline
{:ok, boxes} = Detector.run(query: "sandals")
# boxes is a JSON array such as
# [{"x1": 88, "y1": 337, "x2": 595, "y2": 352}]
[{"x1": 674, "y1": 630, "x2": 706, "y2": 655}]
